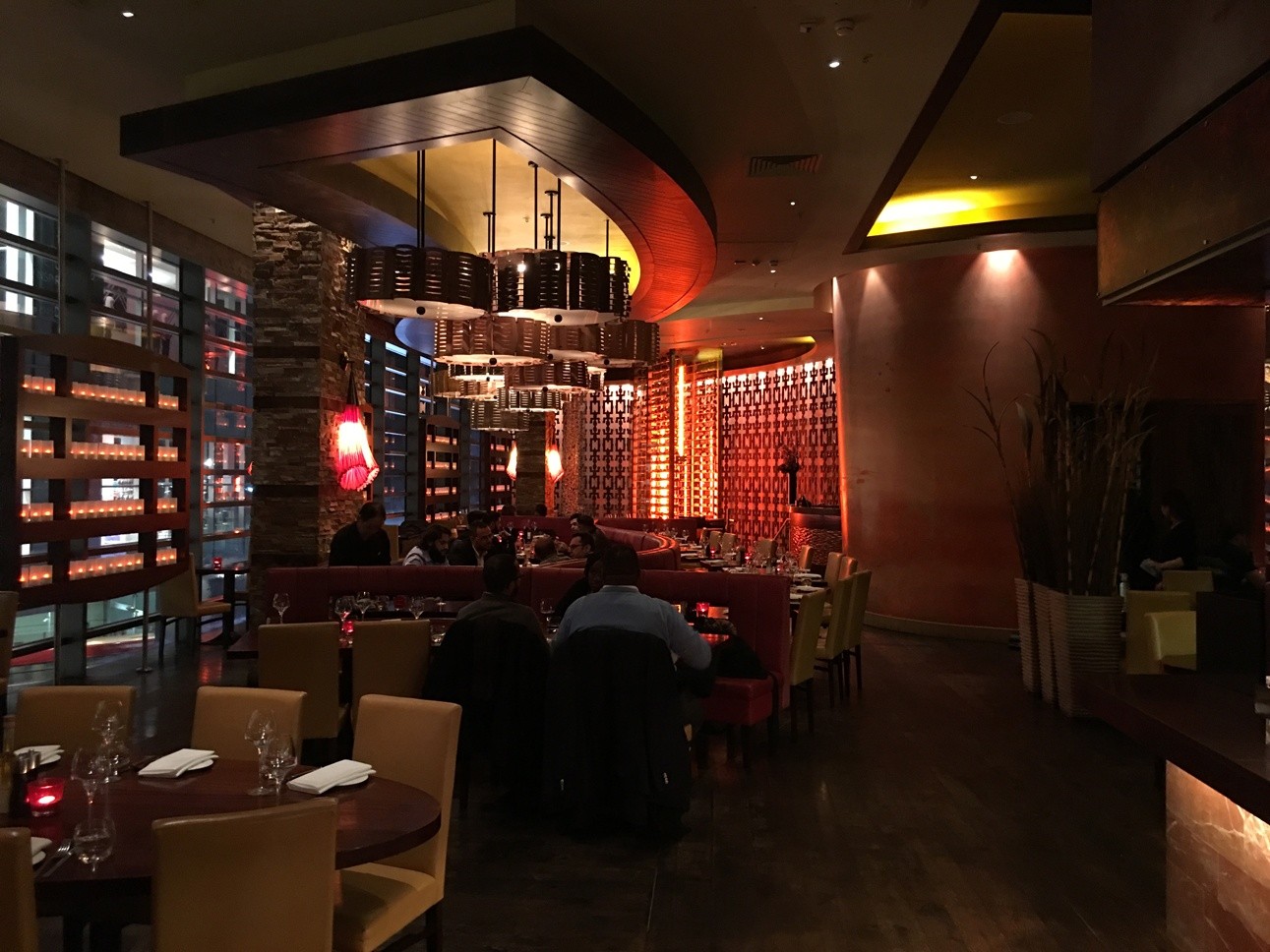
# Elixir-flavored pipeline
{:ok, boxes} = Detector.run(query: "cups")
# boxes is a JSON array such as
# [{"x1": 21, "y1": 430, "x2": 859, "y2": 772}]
[
  {"x1": 213, "y1": 557, "x2": 222, "y2": 570},
  {"x1": 523, "y1": 543, "x2": 531, "y2": 555},
  {"x1": 70, "y1": 818, "x2": 113, "y2": 880},
  {"x1": 327, "y1": 592, "x2": 426, "y2": 622},
  {"x1": 696, "y1": 602, "x2": 709, "y2": 618},
  {"x1": 30, "y1": 777, "x2": 64, "y2": 818},
  {"x1": 429, "y1": 624, "x2": 447, "y2": 647},
  {"x1": 671, "y1": 605, "x2": 681, "y2": 613}
]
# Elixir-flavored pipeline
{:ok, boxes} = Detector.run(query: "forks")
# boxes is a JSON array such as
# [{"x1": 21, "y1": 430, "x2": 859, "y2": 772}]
[{"x1": 33, "y1": 839, "x2": 70, "y2": 877}]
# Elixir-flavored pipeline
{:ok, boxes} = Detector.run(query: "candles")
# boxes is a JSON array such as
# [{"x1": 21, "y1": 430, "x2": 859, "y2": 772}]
[{"x1": 20, "y1": 375, "x2": 180, "y2": 588}]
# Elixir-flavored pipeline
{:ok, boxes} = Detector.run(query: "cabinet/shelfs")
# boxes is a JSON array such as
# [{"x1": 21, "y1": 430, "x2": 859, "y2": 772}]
[
  {"x1": 1, "y1": 141, "x2": 252, "y2": 541},
  {"x1": 0, "y1": 334, "x2": 193, "y2": 609}
]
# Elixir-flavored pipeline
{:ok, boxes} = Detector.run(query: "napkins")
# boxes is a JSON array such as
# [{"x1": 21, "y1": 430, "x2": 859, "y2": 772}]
[
  {"x1": 137, "y1": 747, "x2": 219, "y2": 778},
  {"x1": 286, "y1": 758, "x2": 376, "y2": 797},
  {"x1": 14, "y1": 745, "x2": 65, "y2": 761}
]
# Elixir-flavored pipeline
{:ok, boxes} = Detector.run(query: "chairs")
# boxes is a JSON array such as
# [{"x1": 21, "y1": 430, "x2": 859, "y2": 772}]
[
  {"x1": 0, "y1": 547, "x2": 872, "y2": 952},
  {"x1": 754, "y1": 538, "x2": 780, "y2": 561},
  {"x1": 0, "y1": 590, "x2": 18, "y2": 718},
  {"x1": 698, "y1": 526, "x2": 710, "y2": 547},
  {"x1": 155, "y1": 549, "x2": 231, "y2": 667},
  {"x1": 721, "y1": 532, "x2": 737, "y2": 552},
  {"x1": 708, "y1": 530, "x2": 723, "y2": 551}
]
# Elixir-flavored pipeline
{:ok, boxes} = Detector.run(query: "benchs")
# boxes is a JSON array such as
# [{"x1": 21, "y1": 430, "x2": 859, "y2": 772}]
[{"x1": 268, "y1": 525, "x2": 795, "y2": 773}]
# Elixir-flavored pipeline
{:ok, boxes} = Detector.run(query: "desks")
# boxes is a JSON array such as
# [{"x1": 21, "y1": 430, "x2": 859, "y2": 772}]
[
  {"x1": 0, "y1": 757, "x2": 443, "y2": 952},
  {"x1": 199, "y1": 561, "x2": 252, "y2": 646},
  {"x1": 339, "y1": 619, "x2": 735, "y2": 655}
]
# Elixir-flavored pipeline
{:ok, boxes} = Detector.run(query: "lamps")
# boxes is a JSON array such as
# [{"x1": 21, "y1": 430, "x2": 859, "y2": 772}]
[{"x1": 336, "y1": 149, "x2": 661, "y2": 490}]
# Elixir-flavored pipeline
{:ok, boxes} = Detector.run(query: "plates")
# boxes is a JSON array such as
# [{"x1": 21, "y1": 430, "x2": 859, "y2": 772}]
[
  {"x1": 336, "y1": 775, "x2": 369, "y2": 786},
  {"x1": 187, "y1": 758, "x2": 214, "y2": 770},
  {"x1": 39, "y1": 755, "x2": 61, "y2": 764}
]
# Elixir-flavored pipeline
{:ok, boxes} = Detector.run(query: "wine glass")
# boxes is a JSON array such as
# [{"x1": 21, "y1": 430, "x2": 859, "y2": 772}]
[
  {"x1": 69, "y1": 746, "x2": 108, "y2": 842},
  {"x1": 244, "y1": 707, "x2": 277, "y2": 797},
  {"x1": 540, "y1": 598, "x2": 554, "y2": 633},
  {"x1": 272, "y1": 593, "x2": 289, "y2": 624},
  {"x1": 258, "y1": 732, "x2": 298, "y2": 799},
  {"x1": 91, "y1": 700, "x2": 126, "y2": 784}
]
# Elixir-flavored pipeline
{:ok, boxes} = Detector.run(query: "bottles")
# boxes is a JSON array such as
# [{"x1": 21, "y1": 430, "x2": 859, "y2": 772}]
[
  {"x1": 0, "y1": 744, "x2": 41, "y2": 814},
  {"x1": 642, "y1": 521, "x2": 798, "y2": 575},
  {"x1": 506, "y1": 519, "x2": 537, "y2": 552}
]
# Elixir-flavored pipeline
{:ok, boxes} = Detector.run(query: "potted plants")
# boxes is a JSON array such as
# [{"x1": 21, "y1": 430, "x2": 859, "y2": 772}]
[{"x1": 958, "y1": 328, "x2": 1173, "y2": 718}]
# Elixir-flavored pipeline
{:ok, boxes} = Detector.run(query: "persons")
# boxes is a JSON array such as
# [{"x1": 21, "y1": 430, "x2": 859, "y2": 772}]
[
  {"x1": 1201, "y1": 520, "x2": 1267, "y2": 602},
  {"x1": 328, "y1": 502, "x2": 393, "y2": 566},
  {"x1": 437, "y1": 555, "x2": 557, "y2": 831},
  {"x1": 447, "y1": 503, "x2": 611, "y2": 567},
  {"x1": 545, "y1": 540, "x2": 712, "y2": 846},
  {"x1": 401, "y1": 524, "x2": 452, "y2": 567},
  {"x1": 1153, "y1": 491, "x2": 1200, "y2": 591}
]
